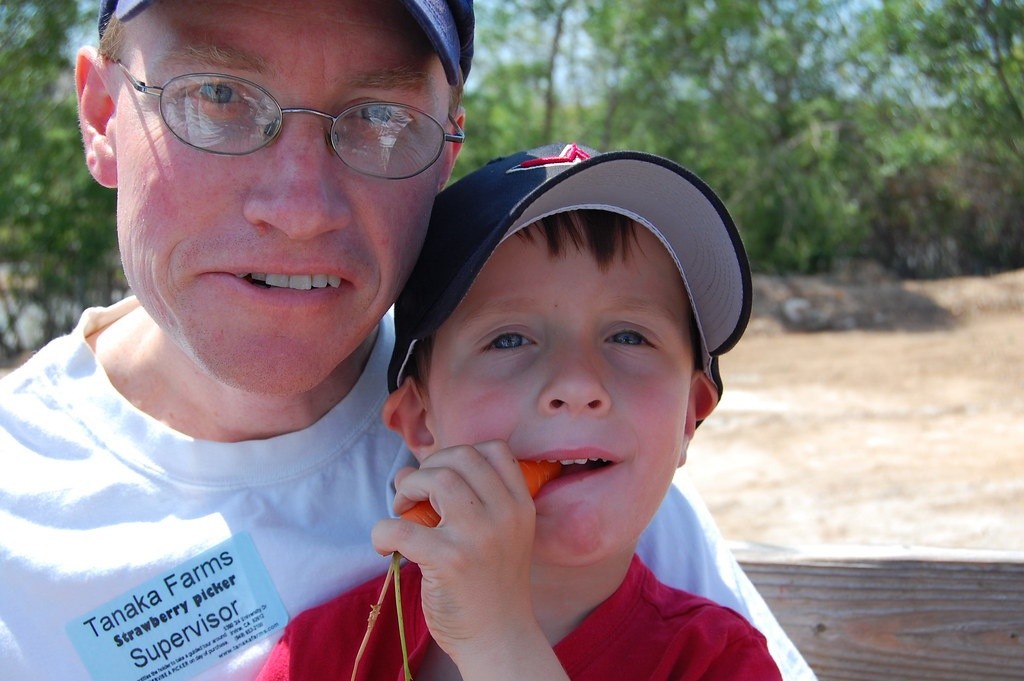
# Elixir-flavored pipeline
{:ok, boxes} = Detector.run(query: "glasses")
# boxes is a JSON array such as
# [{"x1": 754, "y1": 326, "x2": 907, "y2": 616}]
[{"x1": 116, "y1": 59, "x2": 466, "y2": 180}]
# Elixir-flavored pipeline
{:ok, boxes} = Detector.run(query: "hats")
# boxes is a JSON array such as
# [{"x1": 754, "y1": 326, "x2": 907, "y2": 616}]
[
  {"x1": 97, "y1": 0, "x2": 475, "y2": 86},
  {"x1": 386, "y1": 144, "x2": 754, "y2": 431}
]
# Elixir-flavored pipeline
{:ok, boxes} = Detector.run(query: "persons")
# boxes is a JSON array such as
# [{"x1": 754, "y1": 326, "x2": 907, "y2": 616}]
[
  {"x1": 254, "y1": 142, "x2": 785, "y2": 681},
  {"x1": 0, "y1": 0, "x2": 820, "y2": 681}
]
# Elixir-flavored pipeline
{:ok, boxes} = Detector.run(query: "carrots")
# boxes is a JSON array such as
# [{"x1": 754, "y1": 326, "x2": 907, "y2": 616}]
[{"x1": 400, "y1": 459, "x2": 561, "y2": 528}]
